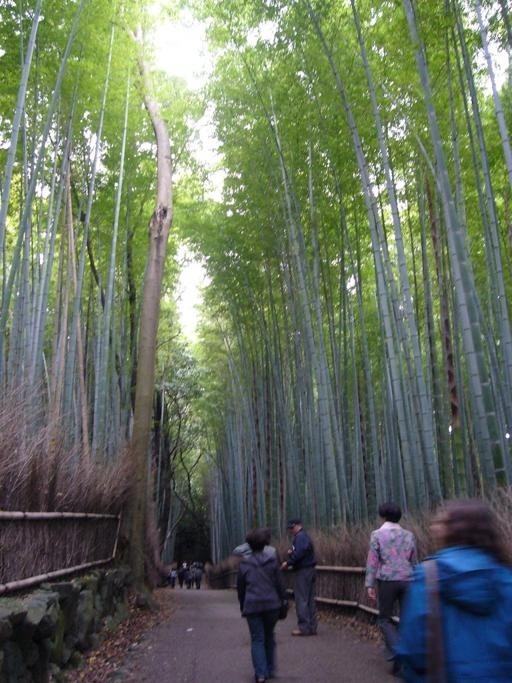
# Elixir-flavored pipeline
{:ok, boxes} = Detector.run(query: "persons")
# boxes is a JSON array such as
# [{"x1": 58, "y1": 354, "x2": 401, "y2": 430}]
[
  {"x1": 362, "y1": 501, "x2": 417, "y2": 674},
  {"x1": 276, "y1": 516, "x2": 319, "y2": 636},
  {"x1": 167, "y1": 558, "x2": 203, "y2": 590},
  {"x1": 395, "y1": 496, "x2": 511, "y2": 682},
  {"x1": 235, "y1": 529, "x2": 285, "y2": 683}
]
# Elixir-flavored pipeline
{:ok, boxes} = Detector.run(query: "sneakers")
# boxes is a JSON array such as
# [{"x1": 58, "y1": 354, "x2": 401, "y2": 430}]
[
  {"x1": 292, "y1": 629, "x2": 317, "y2": 636},
  {"x1": 256, "y1": 677, "x2": 265, "y2": 683}
]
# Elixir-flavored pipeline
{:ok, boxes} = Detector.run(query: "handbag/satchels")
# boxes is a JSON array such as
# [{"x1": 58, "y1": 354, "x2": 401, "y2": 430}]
[{"x1": 279, "y1": 599, "x2": 289, "y2": 619}]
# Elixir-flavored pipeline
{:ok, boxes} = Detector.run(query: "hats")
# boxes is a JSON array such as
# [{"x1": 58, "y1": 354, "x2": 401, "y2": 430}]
[{"x1": 286, "y1": 518, "x2": 301, "y2": 529}]
